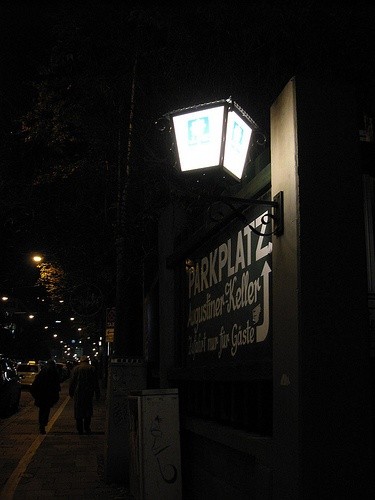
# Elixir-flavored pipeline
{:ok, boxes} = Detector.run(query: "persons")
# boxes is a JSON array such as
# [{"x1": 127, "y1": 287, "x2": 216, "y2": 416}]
[
  {"x1": 69, "y1": 357, "x2": 101, "y2": 434},
  {"x1": 30, "y1": 369, "x2": 62, "y2": 434}
]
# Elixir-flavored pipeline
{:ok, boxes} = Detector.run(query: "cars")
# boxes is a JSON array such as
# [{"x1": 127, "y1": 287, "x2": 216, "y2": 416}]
[
  {"x1": 0, "y1": 354, "x2": 21, "y2": 420},
  {"x1": 15, "y1": 360, "x2": 42, "y2": 388}
]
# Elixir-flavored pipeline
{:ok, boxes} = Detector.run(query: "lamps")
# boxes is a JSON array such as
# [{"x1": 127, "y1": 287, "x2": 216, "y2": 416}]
[{"x1": 161, "y1": 74, "x2": 285, "y2": 240}]
